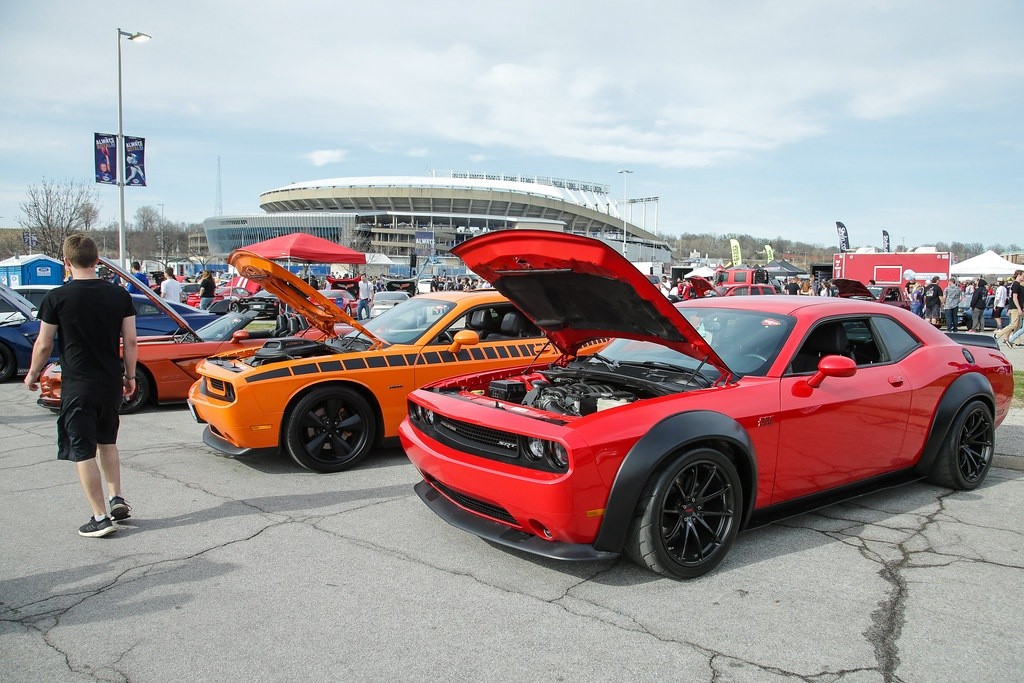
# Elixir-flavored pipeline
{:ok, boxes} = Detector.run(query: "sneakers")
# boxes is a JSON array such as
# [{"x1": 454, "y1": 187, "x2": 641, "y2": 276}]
[
  {"x1": 966, "y1": 329, "x2": 976, "y2": 333},
  {"x1": 1003, "y1": 339, "x2": 1015, "y2": 344},
  {"x1": 109, "y1": 496, "x2": 131, "y2": 521},
  {"x1": 78, "y1": 516, "x2": 115, "y2": 537},
  {"x1": 1015, "y1": 343, "x2": 1024, "y2": 347},
  {"x1": 993, "y1": 329, "x2": 1002, "y2": 333},
  {"x1": 1006, "y1": 341, "x2": 1012, "y2": 348},
  {"x1": 993, "y1": 334, "x2": 998, "y2": 342},
  {"x1": 977, "y1": 329, "x2": 983, "y2": 333},
  {"x1": 946, "y1": 330, "x2": 958, "y2": 333}
]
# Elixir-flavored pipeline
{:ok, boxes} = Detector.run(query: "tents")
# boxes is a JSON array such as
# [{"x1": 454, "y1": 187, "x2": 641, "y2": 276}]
[
  {"x1": 684, "y1": 259, "x2": 807, "y2": 283},
  {"x1": 227, "y1": 233, "x2": 366, "y2": 314},
  {"x1": 950, "y1": 250, "x2": 1024, "y2": 278}
]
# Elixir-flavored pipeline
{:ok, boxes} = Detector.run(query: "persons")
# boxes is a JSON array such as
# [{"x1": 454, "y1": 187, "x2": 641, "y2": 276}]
[
  {"x1": 24, "y1": 234, "x2": 138, "y2": 537},
  {"x1": 777, "y1": 275, "x2": 839, "y2": 298},
  {"x1": 196, "y1": 270, "x2": 215, "y2": 309},
  {"x1": 310, "y1": 271, "x2": 410, "y2": 294},
  {"x1": 430, "y1": 275, "x2": 495, "y2": 315},
  {"x1": 160, "y1": 267, "x2": 183, "y2": 304},
  {"x1": 660, "y1": 275, "x2": 698, "y2": 301},
  {"x1": 357, "y1": 273, "x2": 374, "y2": 320},
  {"x1": 867, "y1": 279, "x2": 875, "y2": 285},
  {"x1": 905, "y1": 270, "x2": 1024, "y2": 349},
  {"x1": 62, "y1": 262, "x2": 149, "y2": 294}
]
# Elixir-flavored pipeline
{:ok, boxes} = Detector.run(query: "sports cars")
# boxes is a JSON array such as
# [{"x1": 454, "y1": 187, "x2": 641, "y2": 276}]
[{"x1": 36, "y1": 254, "x2": 358, "y2": 415}]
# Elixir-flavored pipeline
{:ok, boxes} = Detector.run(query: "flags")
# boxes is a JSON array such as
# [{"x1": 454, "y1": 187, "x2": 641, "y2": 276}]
[
  {"x1": 882, "y1": 230, "x2": 890, "y2": 252},
  {"x1": 730, "y1": 239, "x2": 741, "y2": 267},
  {"x1": 764, "y1": 245, "x2": 774, "y2": 264},
  {"x1": 836, "y1": 221, "x2": 849, "y2": 253}
]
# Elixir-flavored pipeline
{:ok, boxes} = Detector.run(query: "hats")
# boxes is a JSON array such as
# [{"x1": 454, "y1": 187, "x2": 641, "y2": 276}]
[{"x1": 996, "y1": 277, "x2": 1004, "y2": 281}]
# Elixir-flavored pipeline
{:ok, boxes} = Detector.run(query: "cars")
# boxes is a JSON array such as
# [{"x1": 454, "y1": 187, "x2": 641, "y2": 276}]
[
  {"x1": 416, "y1": 278, "x2": 433, "y2": 293},
  {"x1": 397, "y1": 228, "x2": 1016, "y2": 582},
  {"x1": 237, "y1": 287, "x2": 286, "y2": 319},
  {"x1": 186, "y1": 248, "x2": 616, "y2": 474},
  {"x1": 363, "y1": 292, "x2": 428, "y2": 329},
  {"x1": 148, "y1": 270, "x2": 261, "y2": 314},
  {"x1": 0, "y1": 281, "x2": 222, "y2": 385},
  {"x1": 308, "y1": 290, "x2": 358, "y2": 320},
  {"x1": 688, "y1": 275, "x2": 1023, "y2": 329},
  {"x1": 645, "y1": 275, "x2": 660, "y2": 291}
]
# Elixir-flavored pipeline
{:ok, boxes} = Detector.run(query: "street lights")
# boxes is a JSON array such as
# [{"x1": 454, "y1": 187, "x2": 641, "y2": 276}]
[
  {"x1": 29, "y1": 204, "x2": 36, "y2": 255},
  {"x1": 102, "y1": 235, "x2": 107, "y2": 254},
  {"x1": 619, "y1": 169, "x2": 635, "y2": 259},
  {"x1": 116, "y1": 27, "x2": 153, "y2": 286},
  {"x1": 158, "y1": 203, "x2": 164, "y2": 271}
]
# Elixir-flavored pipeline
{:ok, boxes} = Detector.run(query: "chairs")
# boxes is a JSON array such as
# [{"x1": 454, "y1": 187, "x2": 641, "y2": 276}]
[
  {"x1": 792, "y1": 320, "x2": 856, "y2": 374},
  {"x1": 486, "y1": 312, "x2": 527, "y2": 342},
  {"x1": 464, "y1": 309, "x2": 493, "y2": 339},
  {"x1": 268, "y1": 313, "x2": 307, "y2": 337}
]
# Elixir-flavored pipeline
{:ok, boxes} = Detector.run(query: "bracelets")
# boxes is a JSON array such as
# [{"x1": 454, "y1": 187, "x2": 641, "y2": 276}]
[{"x1": 123, "y1": 373, "x2": 136, "y2": 380}]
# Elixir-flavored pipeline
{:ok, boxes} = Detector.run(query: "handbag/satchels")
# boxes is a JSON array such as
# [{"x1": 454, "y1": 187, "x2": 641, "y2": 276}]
[{"x1": 911, "y1": 301, "x2": 918, "y2": 305}]
